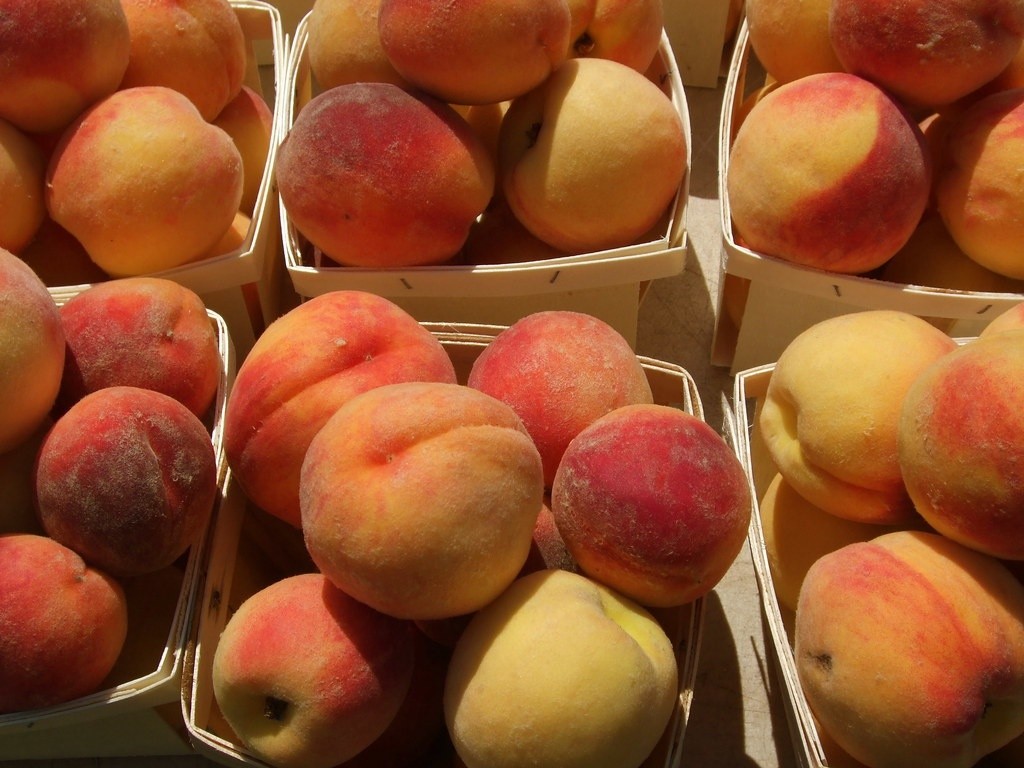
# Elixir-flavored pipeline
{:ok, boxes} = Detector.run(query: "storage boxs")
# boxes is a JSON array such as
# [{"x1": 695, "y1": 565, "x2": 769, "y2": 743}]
[{"x1": 0, "y1": 0, "x2": 1024, "y2": 768}]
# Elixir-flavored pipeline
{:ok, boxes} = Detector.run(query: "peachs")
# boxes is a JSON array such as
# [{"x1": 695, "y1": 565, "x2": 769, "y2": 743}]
[{"x1": 0, "y1": 0, "x2": 1024, "y2": 768}]
